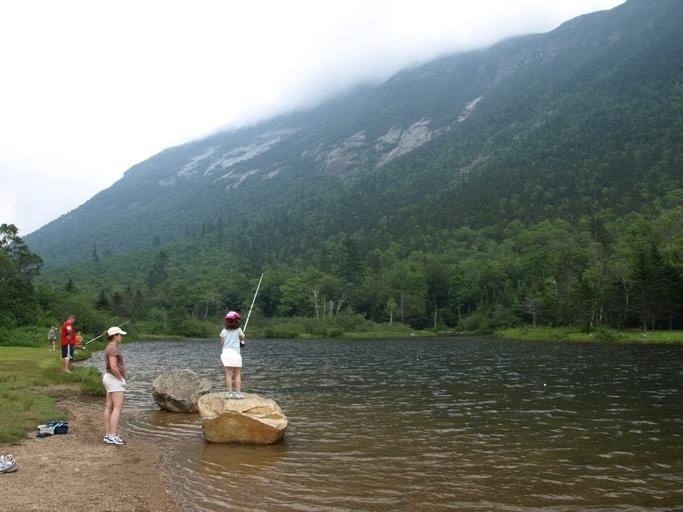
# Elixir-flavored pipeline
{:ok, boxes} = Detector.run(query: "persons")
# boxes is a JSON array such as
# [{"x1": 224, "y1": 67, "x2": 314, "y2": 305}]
[
  {"x1": 60, "y1": 314, "x2": 76, "y2": 374},
  {"x1": 219, "y1": 310, "x2": 246, "y2": 399},
  {"x1": 75, "y1": 331, "x2": 84, "y2": 350},
  {"x1": 101, "y1": 326, "x2": 127, "y2": 444},
  {"x1": 48, "y1": 324, "x2": 57, "y2": 352}
]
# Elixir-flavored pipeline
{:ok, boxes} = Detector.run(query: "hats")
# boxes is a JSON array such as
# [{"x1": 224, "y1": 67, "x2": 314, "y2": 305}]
[{"x1": 106, "y1": 325, "x2": 127, "y2": 337}]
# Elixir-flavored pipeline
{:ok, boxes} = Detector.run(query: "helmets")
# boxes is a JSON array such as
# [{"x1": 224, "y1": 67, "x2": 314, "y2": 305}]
[{"x1": 224, "y1": 310, "x2": 240, "y2": 320}]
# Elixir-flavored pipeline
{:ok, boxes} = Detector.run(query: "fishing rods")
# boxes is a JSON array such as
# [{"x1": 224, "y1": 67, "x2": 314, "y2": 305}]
[
  {"x1": 242, "y1": 273, "x2": 264, "y2": 333},
  {"x1": 84, "y1": 320, "x2": 132, "y2": 345}
]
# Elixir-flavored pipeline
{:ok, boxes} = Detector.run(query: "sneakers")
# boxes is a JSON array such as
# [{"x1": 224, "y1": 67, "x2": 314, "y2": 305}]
[
  {"x1": 103, "y1": 434, "x2": 109, "y2": 443},
  {"x1": 234, "y1": 391, "x2": 245, "y2": 399},
  {"x1": 225, "y1": 392, "x2": 233, "y2": 399},
  {"x1": 0, "y1": 458, "x2": 17, "y2": 473},
  {"x1": 107, "y1": 434, "x2": 126, "y2": 445},
  {"x1": 0, "y1": 455, "x2": 12, "y2": 464}
]
